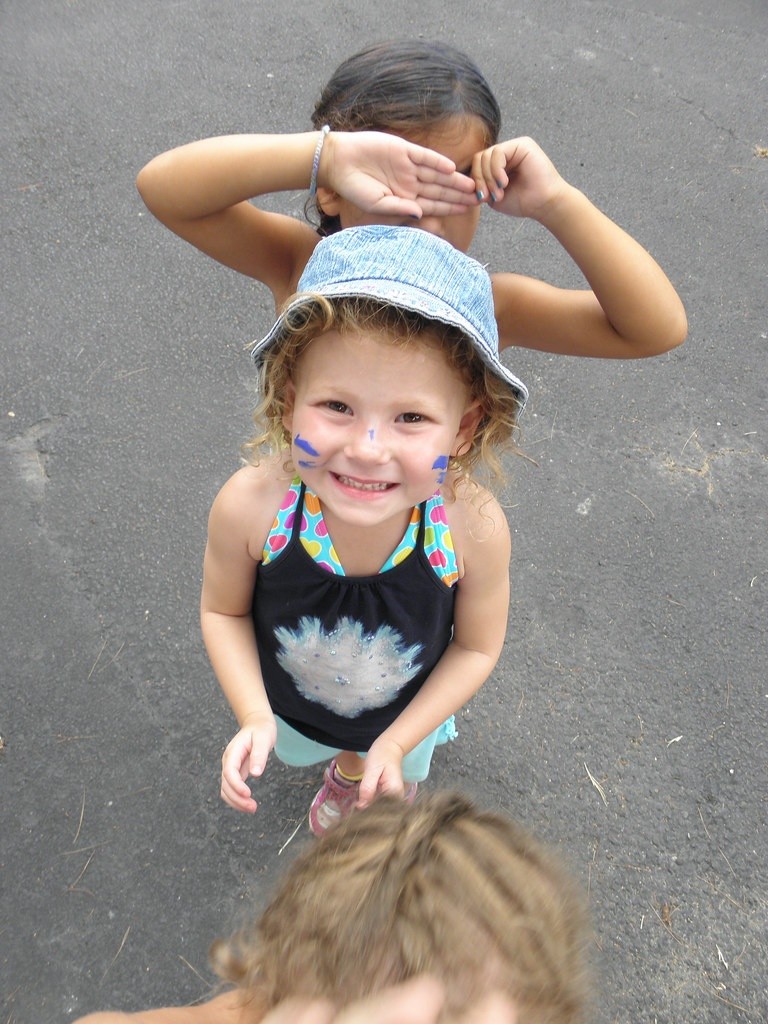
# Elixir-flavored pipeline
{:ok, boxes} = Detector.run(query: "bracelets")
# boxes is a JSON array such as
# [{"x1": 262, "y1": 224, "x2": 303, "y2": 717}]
[{"x1": 309, "y1": 126, "x2": 332, "y2": 199}]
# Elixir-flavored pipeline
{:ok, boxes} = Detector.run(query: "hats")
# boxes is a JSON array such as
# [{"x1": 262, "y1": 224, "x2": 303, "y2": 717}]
[{"x1": 250, "y1": 226, "x2": 530, "y2": 453}]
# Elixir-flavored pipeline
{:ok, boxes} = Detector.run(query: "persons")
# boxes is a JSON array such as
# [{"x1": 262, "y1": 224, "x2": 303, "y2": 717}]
[
  {"x1": 61, "y1": 794, "x2": 590, "y2": 1023},
  {"x1": 198, "y1": 225, "x2": 530, "y2": 814},
  {"x1": 138, "y1": 38, "x2": 690, "y2": 354}
]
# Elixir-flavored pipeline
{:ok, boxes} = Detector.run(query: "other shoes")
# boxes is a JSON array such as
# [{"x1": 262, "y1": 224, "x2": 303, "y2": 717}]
[{"x1": 308, "y1": 759, "x2": 418, "y2": 843}]
